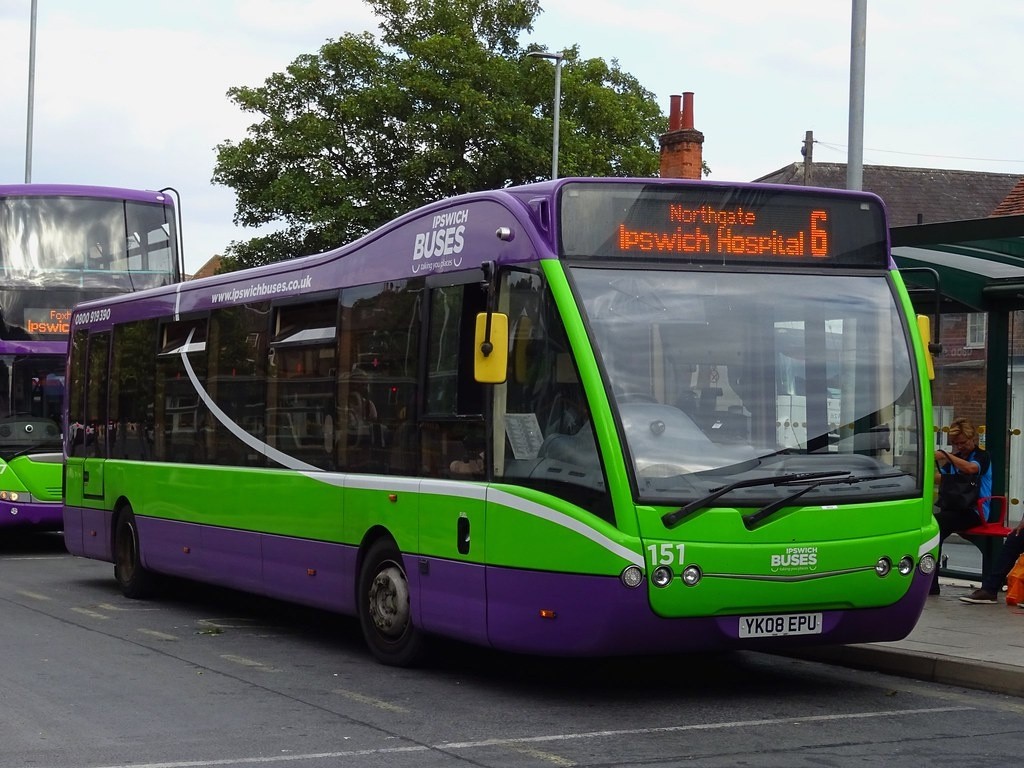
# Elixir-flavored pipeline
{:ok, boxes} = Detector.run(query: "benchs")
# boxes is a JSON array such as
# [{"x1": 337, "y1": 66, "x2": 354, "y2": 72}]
[{"x1": 957, "y1": 495, "x2": 1013, "y2": 587}]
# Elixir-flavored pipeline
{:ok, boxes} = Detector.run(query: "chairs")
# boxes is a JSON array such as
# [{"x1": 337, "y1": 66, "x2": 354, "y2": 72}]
[
  {"x1": 264, "y1": 412, "x2": 323, "y2": 453},
  {"x1": 442, "y1": 429, "x2": 465, "y2": 454}
]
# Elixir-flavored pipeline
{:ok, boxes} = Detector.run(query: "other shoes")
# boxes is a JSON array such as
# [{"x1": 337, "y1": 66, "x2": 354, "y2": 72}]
[{"x1": 928, "y1": 587, "x2": 940, "y2": 596}]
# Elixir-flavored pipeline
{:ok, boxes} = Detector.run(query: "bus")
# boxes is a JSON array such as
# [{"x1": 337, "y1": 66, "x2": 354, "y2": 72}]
[
  {"x1": 62, "y1": 177, "x2": 944, "y2": 670},
  {"x1": 0, "y1": 183, "x2": 185, "y2": 529}
]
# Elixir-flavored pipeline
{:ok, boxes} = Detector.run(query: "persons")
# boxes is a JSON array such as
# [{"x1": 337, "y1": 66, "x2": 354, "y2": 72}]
[
  {"x1": 929, "y1": 416, "x2": 992, "y2": 594},
  {"x1": 959, "y1": 513, "x2": 1024, "y2": 608},
  {"x1": 325, "y1": 370, "x2": 377, "y2": 455},
  {"x1": 544, "y1": 352, "x2": 631, "y2": 440},
  {"x1": 447, "y1": 436, "x2": 487, "y2": 480}
]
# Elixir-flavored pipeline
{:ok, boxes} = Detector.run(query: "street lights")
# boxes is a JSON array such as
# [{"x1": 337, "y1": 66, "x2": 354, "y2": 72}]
[{"x1": 525, "y1": 49, "x2": 563, "y2": 180}]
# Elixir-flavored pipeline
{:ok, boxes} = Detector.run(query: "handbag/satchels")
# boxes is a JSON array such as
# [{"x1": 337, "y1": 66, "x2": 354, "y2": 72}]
[
  {"x1": 1007, "y1": 553, "x2": 1024, "y2": 606},
  {"x1": 936, "y1": 450, "x2": 990, "y2": 511}
]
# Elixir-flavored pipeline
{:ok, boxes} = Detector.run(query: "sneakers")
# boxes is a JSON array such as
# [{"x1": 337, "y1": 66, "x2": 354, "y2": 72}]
[
  {"x1": 958, "y1": 588, "x2": 998, "y2": 603},
  {"x1": 1017, "y1": 601, "x2": 1024, "y2": 607}
]
